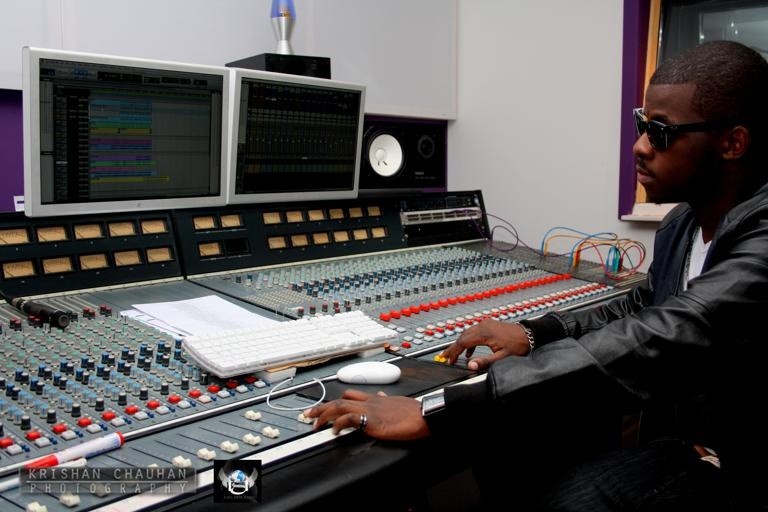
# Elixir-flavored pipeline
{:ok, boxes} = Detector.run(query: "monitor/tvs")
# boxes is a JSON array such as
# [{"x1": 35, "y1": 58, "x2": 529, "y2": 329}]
[
  {"x1": 228, "y1": 67, "x2": 367, "y2": 205},
  {"x1": 21, "y1": 46, "x2": 230, "y2": 218}
]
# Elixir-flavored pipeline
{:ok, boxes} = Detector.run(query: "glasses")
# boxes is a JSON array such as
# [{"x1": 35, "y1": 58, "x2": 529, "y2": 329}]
[{"x1": 633, "y1": 108, "x2": 705, "y2": 152}]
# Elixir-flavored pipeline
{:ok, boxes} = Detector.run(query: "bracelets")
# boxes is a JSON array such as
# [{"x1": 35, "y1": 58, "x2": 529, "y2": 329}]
[{"x1": 514, "y1": 319, "x2": 533, "y2": 355}]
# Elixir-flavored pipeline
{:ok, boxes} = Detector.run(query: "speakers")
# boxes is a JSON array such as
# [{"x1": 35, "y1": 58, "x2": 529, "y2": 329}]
[
  {"x1": 224, "y1": 53, "x2": 331, "y2": 80},
  {"x1": 359, "y1": 113, "x2": 448, "y2": 190}
]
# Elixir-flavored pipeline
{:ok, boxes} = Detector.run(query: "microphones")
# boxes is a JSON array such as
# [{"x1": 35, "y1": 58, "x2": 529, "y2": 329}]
[{"x1": 5, "y1": 295, "x2": 69, "y2": 328}]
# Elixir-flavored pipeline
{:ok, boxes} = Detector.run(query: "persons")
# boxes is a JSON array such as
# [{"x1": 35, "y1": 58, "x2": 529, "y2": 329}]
[{"x1": 305, "y1": 36, "x2": 766, "y2": 508}]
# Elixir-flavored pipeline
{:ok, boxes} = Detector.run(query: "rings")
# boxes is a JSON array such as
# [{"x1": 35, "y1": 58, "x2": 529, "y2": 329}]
[{"x1": 358, "y1": 414, "x2": 367, "y2": 430}]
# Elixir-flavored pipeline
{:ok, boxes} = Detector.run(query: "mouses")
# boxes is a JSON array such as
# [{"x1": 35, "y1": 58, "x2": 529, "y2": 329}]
[{"x1": 337, "y1": 362, "x2": 401, "y2": 385}]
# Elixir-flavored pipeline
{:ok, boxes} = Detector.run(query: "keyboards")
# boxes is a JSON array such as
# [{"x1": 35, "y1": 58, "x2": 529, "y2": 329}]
[{"x1": 180, "y1": 310, "x2": 400, "y2": 381}]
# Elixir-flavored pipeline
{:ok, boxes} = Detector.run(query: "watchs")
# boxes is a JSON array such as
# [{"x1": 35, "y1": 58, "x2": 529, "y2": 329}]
[{"x1": 420, "y1": 392, "x2": 447, "y2": 436}]
